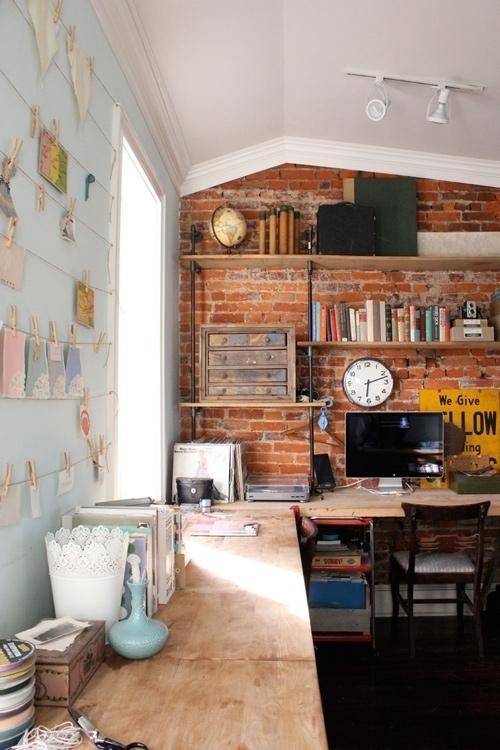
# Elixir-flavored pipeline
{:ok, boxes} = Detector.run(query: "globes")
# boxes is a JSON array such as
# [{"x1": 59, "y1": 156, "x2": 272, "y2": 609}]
[{"x1": 209, "y1": 205, "x2": 249, "y2": 254}]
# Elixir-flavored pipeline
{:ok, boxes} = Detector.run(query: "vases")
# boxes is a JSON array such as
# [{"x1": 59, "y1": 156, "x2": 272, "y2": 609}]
[{"x1": 111, "y1": 578, "x2": 171, "y2": 661}]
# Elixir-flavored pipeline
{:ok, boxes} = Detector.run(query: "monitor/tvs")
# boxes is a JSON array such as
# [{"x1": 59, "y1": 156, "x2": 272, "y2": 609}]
[{"x1": 344, "y1": 411, "x2": 444, "y2": 496}]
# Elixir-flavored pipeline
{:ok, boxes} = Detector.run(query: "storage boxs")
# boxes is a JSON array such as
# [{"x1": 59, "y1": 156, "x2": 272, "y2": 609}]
[{"x1": 14, "y1": 614, "x2": 108, "y2": 707}]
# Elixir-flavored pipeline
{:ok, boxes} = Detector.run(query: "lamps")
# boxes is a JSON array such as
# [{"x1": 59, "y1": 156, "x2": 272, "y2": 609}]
[{"x1": 337, "y1": 66, "x2": 489, "y2": 127}]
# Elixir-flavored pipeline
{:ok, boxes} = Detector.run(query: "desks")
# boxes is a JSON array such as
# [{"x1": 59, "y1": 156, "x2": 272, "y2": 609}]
[
  {"x1": 29, "y1": 508, "x2": 334, "y2": 748},
  {"x1": 223, "y1": 487, "x2": 498, "y2": 658}
]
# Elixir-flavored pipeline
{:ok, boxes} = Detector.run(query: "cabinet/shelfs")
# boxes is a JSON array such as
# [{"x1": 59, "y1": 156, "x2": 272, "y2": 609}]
[
  {"x1": 178, "y1": 246, "x2": 499, "y2": 417},
  {"x1": 197, "y1": 321, "x2": 301, "y2": 403},
  {"x1": 299, "y1": 516, "x2": 375, "y2": 654}
]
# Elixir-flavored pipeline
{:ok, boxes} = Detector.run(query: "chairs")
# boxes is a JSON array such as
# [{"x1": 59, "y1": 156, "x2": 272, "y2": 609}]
[{"x1": 390, "y1": 500, "x2": 494, "y2": 662}]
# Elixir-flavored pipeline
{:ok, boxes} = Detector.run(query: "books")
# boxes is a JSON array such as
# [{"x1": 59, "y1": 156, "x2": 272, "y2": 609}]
[
  {"x1": 191, "y1": 513, "x2": 256, "y2": 534},
  {"x1": 61, "y1": 495, "x2": 185, "y2": 620},
  {"x1": 315, "y1": 546, "x2": 348, "y2": 552},
  {"x1": 313, "y1": 299, "x2": 452, "y2": 344},
  {"x1": 170, "y1": 435, "x2": 247, "y2": 503},
  {"x1": 316, "y1": 540, "x2": 341, "y2": 545},
  {"x1": 189, "y1": 513, "x2": 259, "y2": 537}
]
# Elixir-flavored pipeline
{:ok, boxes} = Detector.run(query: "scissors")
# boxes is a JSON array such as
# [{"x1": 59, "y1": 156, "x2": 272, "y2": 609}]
[{"x1": 67, "y1": 706, "x2": 148, "y2": 750}]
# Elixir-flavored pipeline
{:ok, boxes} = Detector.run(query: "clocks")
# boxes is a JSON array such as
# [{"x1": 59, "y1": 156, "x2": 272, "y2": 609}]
[{"x1": 340, "y1": 355, "x2": 398, "y2": 410}]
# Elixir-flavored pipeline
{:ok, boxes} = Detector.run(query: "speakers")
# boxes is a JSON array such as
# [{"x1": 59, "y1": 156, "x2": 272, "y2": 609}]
[{"x1": 312, "y1": 454, "x2": 336, "y2": 490}]
